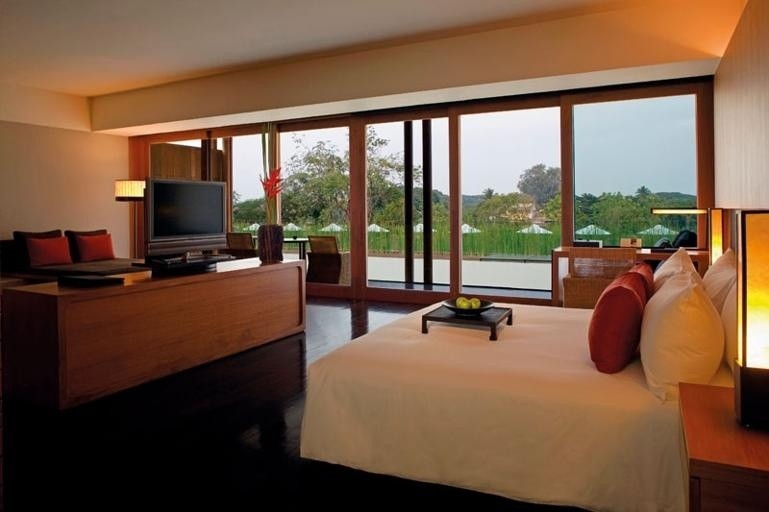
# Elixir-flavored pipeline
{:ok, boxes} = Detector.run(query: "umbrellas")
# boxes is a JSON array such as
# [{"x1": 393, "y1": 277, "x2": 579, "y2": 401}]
[{"x1": 243, "y1": 222, "x2": 263, "y2": 243}]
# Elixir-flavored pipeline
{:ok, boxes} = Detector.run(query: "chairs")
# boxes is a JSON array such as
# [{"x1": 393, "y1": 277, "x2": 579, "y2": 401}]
[
  {"x1": 307, "y1": 235, "x2": 351, "y2": 285},
  {"x1": 226, "y1": 233, "x2": 252, "y2": 251}
]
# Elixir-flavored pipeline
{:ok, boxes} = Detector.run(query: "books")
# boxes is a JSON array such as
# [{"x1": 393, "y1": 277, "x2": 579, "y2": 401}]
[{"x1": 58, "y1": 275, "x2": 124, "y2": 288}]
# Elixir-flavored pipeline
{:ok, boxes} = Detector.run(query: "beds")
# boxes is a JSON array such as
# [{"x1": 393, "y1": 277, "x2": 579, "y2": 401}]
[{"x1": 302, "y1": 206, "x2": 768, "y2": 509}]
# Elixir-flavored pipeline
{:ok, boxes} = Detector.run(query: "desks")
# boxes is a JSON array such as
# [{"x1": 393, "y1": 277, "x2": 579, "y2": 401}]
[
  {"x1": 422, "y1": 304, "x2": 513, "y2": 341},
  {"x1": 549, "y1": 244, "x2": 707, "y2": 308},
  {"x1": 280, "y1": 237, "x2": 311, "y2": 260},
  {"x1": 1, "y1": 257, "x2": 307, "y2": 410}
]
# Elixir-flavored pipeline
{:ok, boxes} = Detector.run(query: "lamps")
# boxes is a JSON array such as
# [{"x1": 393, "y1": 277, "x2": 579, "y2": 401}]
[
  {"x1": 735, "y1": 207, "x2": 766, "y2": 432},
  {"x1": 114, "y1": 180, "x2": 148, "y2": 259},
  {"x1": 649, "y1": 203, "x2": 710, "y2": 251},
  {"x1": 709, "y1": 207, "x2": 730, "y2": 264}
]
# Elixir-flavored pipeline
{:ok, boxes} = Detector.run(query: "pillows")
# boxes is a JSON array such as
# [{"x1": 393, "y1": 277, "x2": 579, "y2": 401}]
[
  {"x1": 25, "y1": 238, "x2": 72, "y2": 267},
  {"x1": 14, "y1": 228, "x2": 62, "y2": 275},
  {"x1": 65, "y1": 229, "x2": 109, "y2": 266},
  {"x1": 73, "y1": 233, "x2": 116, "y2": 262},
  {"x1": 588, "y1": 244, "x2": 742, "y2": 401}
]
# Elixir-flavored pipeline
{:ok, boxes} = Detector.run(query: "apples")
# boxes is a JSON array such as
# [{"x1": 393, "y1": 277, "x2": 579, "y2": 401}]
[{"x1": 456, "y1": 297, "x2": 480, "y2": 309}]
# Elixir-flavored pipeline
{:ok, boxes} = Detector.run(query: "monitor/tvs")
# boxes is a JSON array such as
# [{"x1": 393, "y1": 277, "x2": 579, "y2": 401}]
[{"x1": 143, "y1": 177, "x2": 228, "y2": 262}]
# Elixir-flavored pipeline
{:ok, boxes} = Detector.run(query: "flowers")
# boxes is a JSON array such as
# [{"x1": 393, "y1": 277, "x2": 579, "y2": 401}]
[{"x1": 260, "y1": 166, "x2": 284, "y2": 225}]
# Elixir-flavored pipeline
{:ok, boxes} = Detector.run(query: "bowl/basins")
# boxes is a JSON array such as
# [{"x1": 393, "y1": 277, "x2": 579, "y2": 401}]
[{"x1": 441, "y1": 299, "x2": 494, "y2": 317}]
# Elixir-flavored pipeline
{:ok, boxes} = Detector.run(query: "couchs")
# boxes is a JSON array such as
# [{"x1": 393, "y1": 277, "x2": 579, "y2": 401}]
[{"x1": 0, "y1": 239, "x2": 147, "y2": 285}]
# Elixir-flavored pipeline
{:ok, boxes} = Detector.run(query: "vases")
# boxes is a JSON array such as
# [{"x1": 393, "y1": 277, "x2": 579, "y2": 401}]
[{"x1": 259, "y1": 224, "x2": 285, "y2": 262}]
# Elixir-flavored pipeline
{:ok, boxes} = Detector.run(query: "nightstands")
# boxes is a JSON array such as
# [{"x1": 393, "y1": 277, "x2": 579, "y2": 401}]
[{"x1": 676, "y1": 382, "x2": 769, "y2": 510}]
